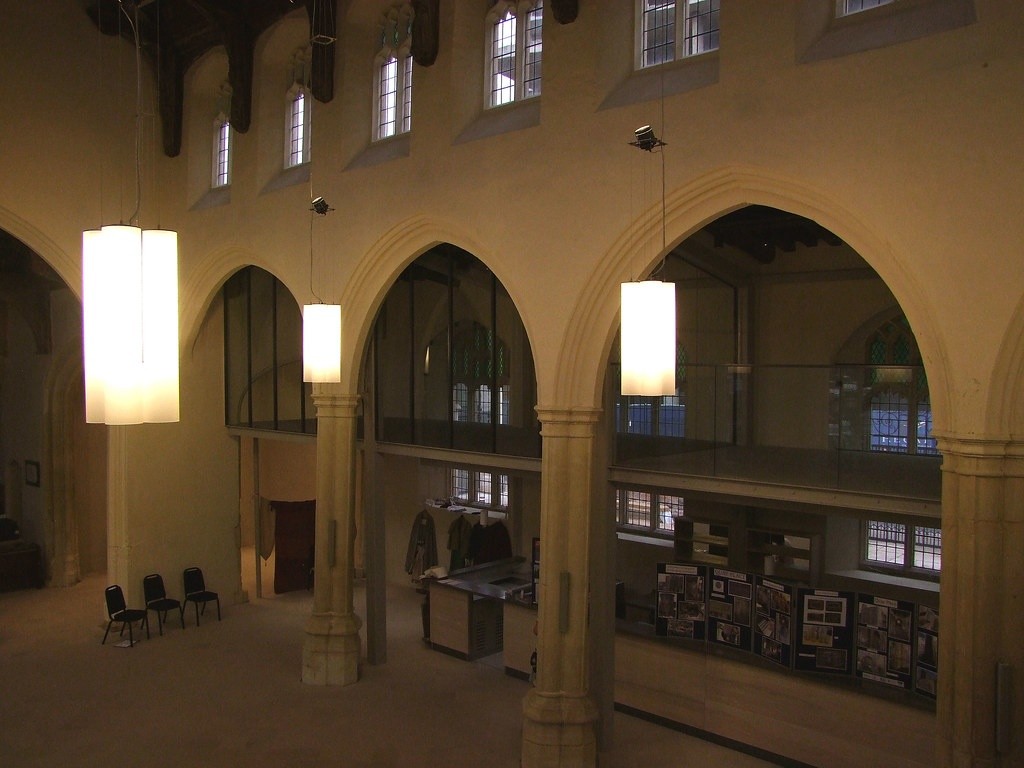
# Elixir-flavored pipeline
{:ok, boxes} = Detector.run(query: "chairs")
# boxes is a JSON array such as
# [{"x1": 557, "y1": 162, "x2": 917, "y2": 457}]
[
  {"x1": 181, "y1": 566, "x2": 225, "y2": 626},
  {"x1": 140, "y1": 573, "x2": 185, "y2": 635},
  {"x1": 99, "y1": 585, "x2": 150, "y2": 648}
]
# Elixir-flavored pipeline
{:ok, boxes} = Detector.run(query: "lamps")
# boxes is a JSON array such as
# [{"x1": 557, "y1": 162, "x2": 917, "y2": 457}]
[
  {"x1": 85, "y1": 0, "x2": 184, "y2": 424},
  {"x1": 302, "y1": 0, "x2": 343, "y2": 383},
  {"x1": 621, "y1": 4, "x2": 676, "y2": 398},
  {"x1": 309, "y1": 195, "x2": 336, "y2": 217},
  {"x1": 628, "y1": 127, "x2": 668, "y2": 156}
]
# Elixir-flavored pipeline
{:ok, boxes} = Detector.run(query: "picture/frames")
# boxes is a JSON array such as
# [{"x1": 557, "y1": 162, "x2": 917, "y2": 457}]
[{"x1": 25, "y1": 461, "x2": 40, "y2": 485}]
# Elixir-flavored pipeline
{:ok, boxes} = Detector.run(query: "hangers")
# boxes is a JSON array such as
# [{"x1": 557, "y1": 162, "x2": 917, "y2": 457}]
[
  {"x1": 456, "y1": 507, "x2": 473, "y2": 520},
  {"x1": 420, "y1": 503, "x2": 432, "y2": 518}
]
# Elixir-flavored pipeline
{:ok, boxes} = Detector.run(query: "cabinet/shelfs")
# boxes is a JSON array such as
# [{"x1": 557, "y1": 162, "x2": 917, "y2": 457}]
[{"x1": 669, "y1": 515, "x2": 821, "y2": 585}]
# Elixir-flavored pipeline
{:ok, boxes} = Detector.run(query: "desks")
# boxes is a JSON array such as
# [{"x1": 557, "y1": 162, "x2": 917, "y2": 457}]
[{"x1": 0, "y1": 539, "x2": 41, "y2": 593}]
